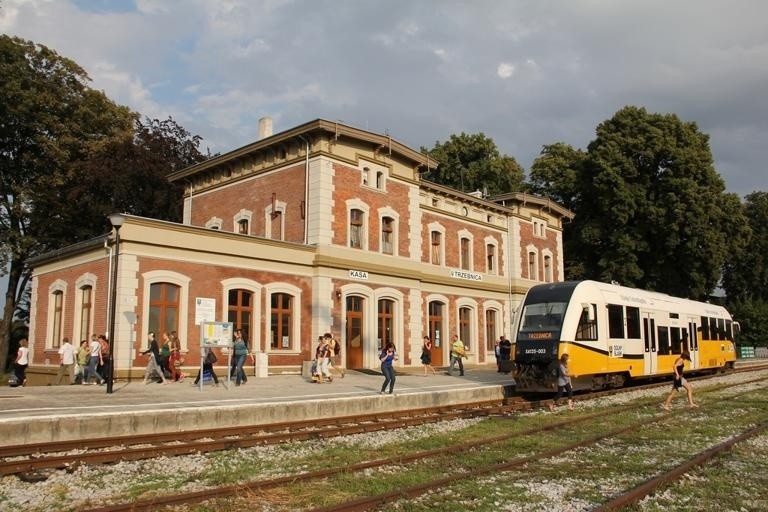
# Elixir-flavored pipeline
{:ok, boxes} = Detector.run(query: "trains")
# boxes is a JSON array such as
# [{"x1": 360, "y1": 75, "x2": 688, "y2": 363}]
[{"x1": 509, "y1": 280, "x2": 745, "y2": 396}]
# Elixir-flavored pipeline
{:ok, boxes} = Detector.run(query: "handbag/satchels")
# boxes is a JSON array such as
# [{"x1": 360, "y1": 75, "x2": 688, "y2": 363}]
[
  {"x1": 85, "y1": 355, "x2": 90, "y2": 363},
  {"x1": 204, "y1": 351, "x2": 217, "y2": 366}
]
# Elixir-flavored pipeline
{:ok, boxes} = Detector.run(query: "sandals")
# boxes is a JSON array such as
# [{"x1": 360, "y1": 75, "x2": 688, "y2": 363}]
[
  {"x1": 548, "y1": 404, "x2": 555, "y2": 412},
  {"x1": 22, "y1": 379, "x2": 27, "y2": 387}
]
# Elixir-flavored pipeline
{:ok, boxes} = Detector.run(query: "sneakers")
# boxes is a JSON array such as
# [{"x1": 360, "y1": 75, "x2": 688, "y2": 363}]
[
  {"x1": 329, "y1": 377, "x2": 333, "y2": 382},
  {"x1": 179, "y1": 375, "x2": 184, "y2": 383},
  {"x1": 100, "y1": 380, "x2": 105, "y2": 386}
]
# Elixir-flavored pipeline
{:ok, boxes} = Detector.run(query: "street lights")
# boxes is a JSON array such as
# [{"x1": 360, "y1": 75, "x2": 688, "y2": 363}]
[{"x1": 103, "y1": 213, "x2": 131, "y2": 394}]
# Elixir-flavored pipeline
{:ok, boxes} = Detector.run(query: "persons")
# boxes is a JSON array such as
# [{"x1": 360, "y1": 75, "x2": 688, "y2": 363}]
[
  {"x1": 420, "y1": 336, "x2": 437, "y2": 377},
  {"x1": 13, "y1": 339, "x2": 29, "y2": 387},
  {"x1": 494, "y1": 340, "x2": 501, "y2": 365},
  {"x1": 443, "y1": 334, "x2": 466, "y2": 377},
  {"x1": 379, "y1": 342, "x2": 399, "y2": 395},
  {"x1": 498, "y1": 336, "x2": 512, "y2": 375},
  {"x1": 547, "y1": 353, "x2": 578, "y2": 413},
  {"x1": 54, "y1": 328, "x2": 346, "y2": 390},
  {"x1": 663, "y1": 350, "x2": 700, "y2": 412}
]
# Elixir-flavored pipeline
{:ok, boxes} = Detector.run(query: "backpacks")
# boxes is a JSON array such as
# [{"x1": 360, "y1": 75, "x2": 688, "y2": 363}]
[{"x1": 329, "y1": 338, "x2": 341, "y2": 355}]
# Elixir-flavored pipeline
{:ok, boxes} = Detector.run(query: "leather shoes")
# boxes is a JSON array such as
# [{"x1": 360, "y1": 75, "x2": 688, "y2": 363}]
[
  {"x1": 342, "y1": 371, "x2": 346, "y2": 378},
  {"x1": 236, "y1": 383, "x2": 240, "y2": 387},
  {"x1": 242, "y1": 380, "x2": 247, "y2": 384}
]
